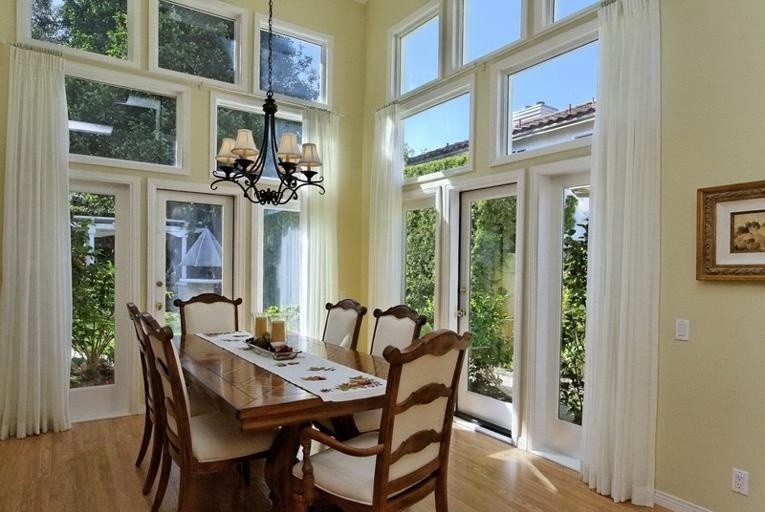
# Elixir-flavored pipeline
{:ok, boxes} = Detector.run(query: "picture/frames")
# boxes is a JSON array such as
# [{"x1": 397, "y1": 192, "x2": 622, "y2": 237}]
[{"x1": 696, "y1": 180, "x2": 765, "y2": 281}]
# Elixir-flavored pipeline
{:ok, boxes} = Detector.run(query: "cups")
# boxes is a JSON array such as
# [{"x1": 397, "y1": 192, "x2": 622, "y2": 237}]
[
  {"x1": 270, "y1": 320, "x2": 287, "y2": 343},
  {"x1": 254, "y1": 312, "x2": 268, "y2": 343}
]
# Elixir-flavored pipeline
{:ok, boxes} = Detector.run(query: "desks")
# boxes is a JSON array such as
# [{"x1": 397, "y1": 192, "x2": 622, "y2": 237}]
[{"x1": 171, "y1": 331, "x2": 391, "y2": 511}]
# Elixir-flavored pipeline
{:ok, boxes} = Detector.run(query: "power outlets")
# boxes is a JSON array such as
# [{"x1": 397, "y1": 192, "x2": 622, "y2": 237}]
[{"x1": 731, "y1": 468, "x2": 749, "y2": 496}]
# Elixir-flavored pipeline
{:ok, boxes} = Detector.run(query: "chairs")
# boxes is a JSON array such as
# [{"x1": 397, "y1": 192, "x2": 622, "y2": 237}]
[
  {"x1": 173, "y1": 292, "x2": 242, "y2": 385},
  {"x1": 313, "y1": 305, "x2": 427, "y2": 442},
  {"x1": 127, "y1": 302, "x2": 162, "y2": 495},
  {"x1": 141, "y1": 311, "x2": 277, "y2": 512},
  {"x1": 282, "y1": 329, "x2": 473, "y2": 512},
  {"x1": 313, "y1": 298, "x2": 367, "y2": 350}
]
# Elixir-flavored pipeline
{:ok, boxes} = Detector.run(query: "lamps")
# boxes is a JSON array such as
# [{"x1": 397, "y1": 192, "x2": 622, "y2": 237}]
[{"x1": 211, "y1": 0, "x2": 326, "y2": 205}]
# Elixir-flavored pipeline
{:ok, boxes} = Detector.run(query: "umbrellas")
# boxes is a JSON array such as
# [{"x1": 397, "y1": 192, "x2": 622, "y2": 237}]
[{"x1": 179, "y1": 225, "x2": 222, "y2": 267}]
